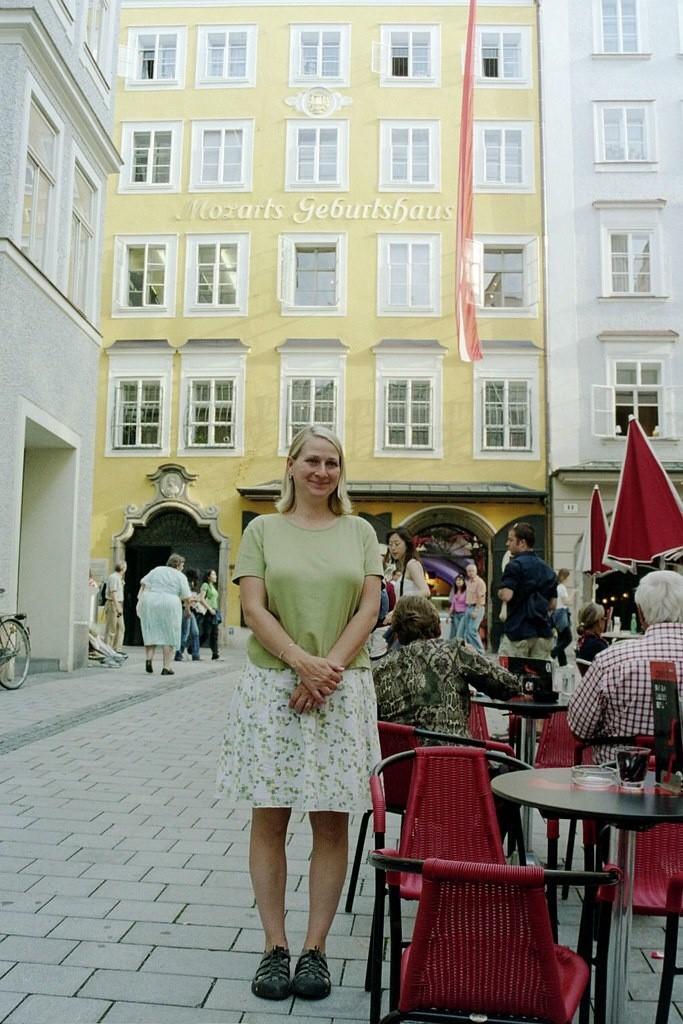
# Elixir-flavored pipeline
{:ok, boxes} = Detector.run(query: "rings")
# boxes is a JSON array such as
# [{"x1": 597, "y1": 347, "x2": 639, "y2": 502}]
[{"x1": 306, "y1": 701, "x2": 312, "y2": 704}]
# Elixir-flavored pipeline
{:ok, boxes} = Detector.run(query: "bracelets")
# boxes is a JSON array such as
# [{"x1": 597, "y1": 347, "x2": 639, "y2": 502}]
[{"x1": 279, "y1": 643, "x2": 295, "y2": 659}]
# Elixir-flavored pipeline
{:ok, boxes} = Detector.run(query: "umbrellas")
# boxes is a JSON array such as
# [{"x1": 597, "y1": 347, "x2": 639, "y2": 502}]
[
  {"x1": 582, "y1": 483, "x2": 612, "y2": 578},
  {"x1": 606, "y1": 415, "x2": 683, "y2": 574}
]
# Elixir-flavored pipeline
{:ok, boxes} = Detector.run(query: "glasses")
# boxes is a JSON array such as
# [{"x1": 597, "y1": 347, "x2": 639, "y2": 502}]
[
  {"x1": 387, "y1": 542, "x2": 401, "y2": 549},
  {"x1": 601, "y1": 617, "x2": 608, "y2": 621}
]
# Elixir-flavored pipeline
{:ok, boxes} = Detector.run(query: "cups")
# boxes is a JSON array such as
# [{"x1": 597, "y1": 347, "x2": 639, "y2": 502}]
[
  {"x1": 561, "y1": 671, "x2": 578, "y2": 697},
  {"x1": 613, "y1": 746, "x2": 651, "y2": 789},
  {"x1": 522, "y1": 676, "x2": 544, "y2": 699}
]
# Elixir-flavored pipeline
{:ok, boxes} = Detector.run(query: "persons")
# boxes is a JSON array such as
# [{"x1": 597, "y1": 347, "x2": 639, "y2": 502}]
[
  {"x1": 551, "y1": 568, "x2": 578, "y2": 666},
  {"x1": 576, "y1": 602, "x2": 609, "y2": 669},
  {"x1": 215, "y1": 426, "x2": 387, "y2": 1001},
  {"x1": 445, "y1": 566, "x2": 486, "y2": 654},
  {"x1": 370, "y1": 528, "x2": 431, "y2": 658},
  {"x1": 137, "y1": 554, "x2": 192, "y2": 675},
  {"x1": 88, "y1": 561, "x2": 129, "y2": 667},
  {"x1": 370, "y1": 595, "x2": 523, "y2": 803},
  {"x1": 175, "y1": 569, "x2": 221, "y2": 661},
  {"x1": 497, "y1": 524, "x2": 558, "y2": 741},
  {"x1": 565, "y1": 571, "x2": 683, "y2": 763},
  {"x1": 165, "y1": 477, "x2": 179, "y2": 495}
]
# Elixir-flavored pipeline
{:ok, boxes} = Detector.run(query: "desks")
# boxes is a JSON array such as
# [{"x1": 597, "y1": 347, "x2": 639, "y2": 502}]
[
  {"x1": 470, "y1": 697, "x2": 572, "y2": 868},
  {"x1": 490, "y1": 767, "x2": 683, "y2": 1024}
]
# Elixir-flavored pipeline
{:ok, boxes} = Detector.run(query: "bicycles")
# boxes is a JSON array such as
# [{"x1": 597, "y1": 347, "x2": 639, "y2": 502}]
[{"x1": 0, "y1": 587, "x2": 32, "y2": 691}]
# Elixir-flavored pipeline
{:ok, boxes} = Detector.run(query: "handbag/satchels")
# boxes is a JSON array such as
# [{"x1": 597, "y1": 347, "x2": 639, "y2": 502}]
[
  {"x1": 212, "y1": 610, "x2": 222, "y2": 625},
  {"x1": 98, "y1": 582, "x2": 107, "y2": 606}
]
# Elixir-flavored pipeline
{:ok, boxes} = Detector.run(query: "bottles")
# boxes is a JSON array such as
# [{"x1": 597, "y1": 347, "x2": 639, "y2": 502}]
[{"x1": 630, "y1": 613, "x2": 637, "y2": 633}]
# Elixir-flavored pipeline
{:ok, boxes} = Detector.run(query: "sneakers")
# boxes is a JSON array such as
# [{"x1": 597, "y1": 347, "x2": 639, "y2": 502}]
[
  {"x1": 292, "y1": 945, "x2": 331, "y2": 1002},
  {"x1": 251, "y1": 945, "x2": 291, "y2": 1001}
]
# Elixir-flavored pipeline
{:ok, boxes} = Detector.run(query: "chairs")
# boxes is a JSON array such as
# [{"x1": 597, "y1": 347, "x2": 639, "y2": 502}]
[{"x1": 346, "y1": 658, "x2": 683, "y2": 1024}]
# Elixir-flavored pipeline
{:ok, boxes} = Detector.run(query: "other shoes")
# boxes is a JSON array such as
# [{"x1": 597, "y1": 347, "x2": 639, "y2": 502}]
[
  {"x1": 193, "y1": 656, "x2": 202, "y2": 661},
  {"x1": 560, "y1": 663, "x2": 573, "y2": 669},
  {"x1": 212, "y1": 653, "x2": 219, "y2": 660},
  {"x1": 161, "y1": 668, "x2": 174, "y2": 675},
  {"x1": 187, "y1": 645, "x2": 193, "y2": 654},
  {"x1": 145, "y1": 660, "x2": 153, "y2": 673},
  {"x1": 117, "y1": 652, "x2": 128, "y2": 660},
  {"x1": 89, "y1": 653, "x2": 105, "y2": 660},
  {"x1": 175, "y1": 652, "x2": 182, "y2": 661}
]
